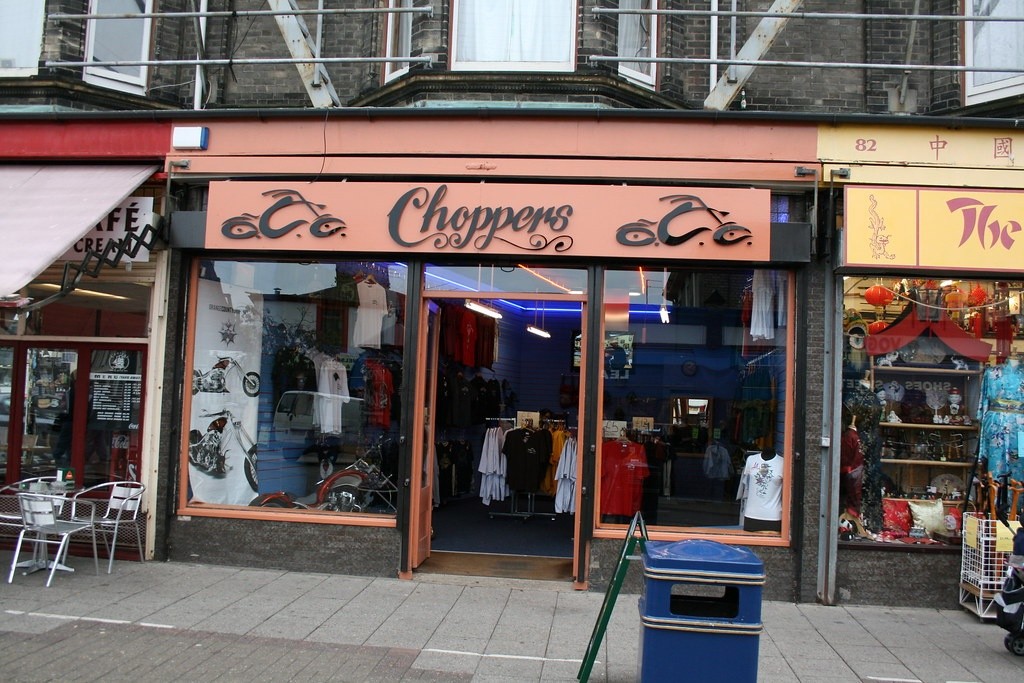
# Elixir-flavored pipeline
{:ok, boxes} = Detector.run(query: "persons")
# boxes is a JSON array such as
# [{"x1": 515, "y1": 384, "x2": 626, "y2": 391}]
[
  {"x1": 876, "y1": 387, "x2": 886, "y2": 420},
  {"x1": 949, "y1": 389, "x2": 964, "y2": 415},
  {"x1": 977, "y1": 358, "x2": 1024, "y2": 485},
  {"x1": 743, "y1": 447, "x2": 783, "y2": 521},
  {"x1": 605, "y1": 341, "x2": 627, "y2": 369},
  {"x1": 842, "y1": 425, "x2": 865, "y2": 516}
]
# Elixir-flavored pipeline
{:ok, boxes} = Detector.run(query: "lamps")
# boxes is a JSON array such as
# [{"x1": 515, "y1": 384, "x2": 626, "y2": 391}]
[
  {"x1": 527, "y1": 289, "x2": 551, "y2": 338},
  {"x1": 465, "y1": 264, "x2": 503, "y2": 318},
  {"x1": 659, "y1": 268, "x2": 670, "y2": 324}
]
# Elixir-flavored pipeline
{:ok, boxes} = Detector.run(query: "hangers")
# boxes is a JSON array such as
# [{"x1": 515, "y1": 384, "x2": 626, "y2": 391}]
[
  {"x1": 353, "y1": 265, "x2": 376, "y2": 284},
  {"x1": 485, "y1": 416, "x2": 564, "y2": 431},
  {"x1": 618, "y1": 428, "x2": 655, "y2": 444}
]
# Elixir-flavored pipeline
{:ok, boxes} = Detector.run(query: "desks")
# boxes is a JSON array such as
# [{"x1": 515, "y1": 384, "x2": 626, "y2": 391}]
[{"x1": 8, "y1": 482, "x2": 85, "y2": 575}]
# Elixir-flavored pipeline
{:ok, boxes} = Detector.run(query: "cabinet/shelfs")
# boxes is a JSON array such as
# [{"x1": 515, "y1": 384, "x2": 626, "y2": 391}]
[{"x1": 870, "y1": 356, "x2": 980, "y2": 466}]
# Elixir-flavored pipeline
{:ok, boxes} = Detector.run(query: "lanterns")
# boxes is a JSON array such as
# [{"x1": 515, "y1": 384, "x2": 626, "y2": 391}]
[
  {"x1": 867, "y1": 322, "x2": 890, "y2": 336},
  {"x1": 945, "y1": 291, "x2": 963, "y2": 312},
  {"x1": 865, "y1": 285, "x2": 895, "y2": 307}
]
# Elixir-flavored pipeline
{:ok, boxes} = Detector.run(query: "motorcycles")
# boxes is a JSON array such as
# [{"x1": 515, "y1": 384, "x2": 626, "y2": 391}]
[
  {"x1": 193, "y1": 349, "x2": 260, "y2": 398},
  {"x1": 248, "y1": 435, "x2": 439, "y2": 541},
  {"x1": 189, "y1": 407, "x2": 259, "y2": 492}
]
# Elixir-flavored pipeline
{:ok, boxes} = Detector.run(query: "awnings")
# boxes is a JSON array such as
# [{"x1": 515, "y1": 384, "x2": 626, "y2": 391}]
[{"x1": 1, "y1": 165, "x2": 159, "y2": 307}]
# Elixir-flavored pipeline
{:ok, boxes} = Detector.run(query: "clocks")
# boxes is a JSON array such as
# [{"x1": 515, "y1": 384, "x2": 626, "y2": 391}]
[{"x1": 682, "y1": 361, "x2": 698, "y2": 376}]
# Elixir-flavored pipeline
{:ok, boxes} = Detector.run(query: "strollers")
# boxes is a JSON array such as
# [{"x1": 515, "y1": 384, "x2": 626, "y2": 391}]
[{"x1": 992, "y1": 472, "x2": 1024, "y2": 657}]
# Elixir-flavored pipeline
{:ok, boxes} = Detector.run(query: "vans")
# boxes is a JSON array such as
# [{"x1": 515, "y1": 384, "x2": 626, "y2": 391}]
[{"x1": 272, "y1": 389, "x2": 367, "y2": 462}]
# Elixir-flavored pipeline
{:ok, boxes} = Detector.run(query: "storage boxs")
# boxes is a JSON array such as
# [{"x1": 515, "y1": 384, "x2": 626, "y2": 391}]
[{"x1": 57, "y1": 468, "x2": 76, "y2": 490}]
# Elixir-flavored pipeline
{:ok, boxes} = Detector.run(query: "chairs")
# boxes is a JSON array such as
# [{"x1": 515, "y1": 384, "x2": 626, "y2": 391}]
[{"x1": 0, "y1": 476, "x2": 143, "y2": 587}]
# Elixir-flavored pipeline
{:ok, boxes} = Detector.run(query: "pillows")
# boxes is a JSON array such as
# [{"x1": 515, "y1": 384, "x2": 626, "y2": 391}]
[
  {"x1": 908, "y1": 498, "x2": 948, "y2": 537},
  {"x1": 881, "y1": 499, "x2": 914, "y2": 538}
]
